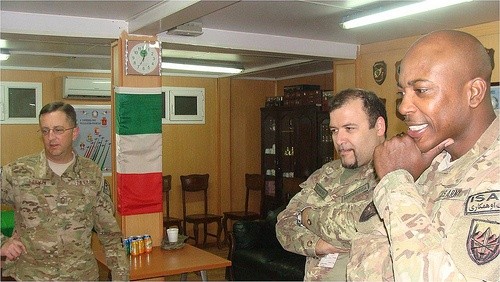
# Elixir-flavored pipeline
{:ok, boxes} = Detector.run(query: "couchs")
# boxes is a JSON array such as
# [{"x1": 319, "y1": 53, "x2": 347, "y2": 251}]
[{"x1": 224, "y1": 207, "x2": 306, "y2": 281}]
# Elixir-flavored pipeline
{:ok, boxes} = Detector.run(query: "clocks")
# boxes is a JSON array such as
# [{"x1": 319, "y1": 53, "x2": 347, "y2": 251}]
[{"x1": 125, "y1": 39, "x2": 162, "y2": 76}]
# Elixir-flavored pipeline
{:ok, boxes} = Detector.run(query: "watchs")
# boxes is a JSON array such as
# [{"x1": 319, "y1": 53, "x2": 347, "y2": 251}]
[{"x1": 296, "y1": 204, "x2": 313, "y2": 227}]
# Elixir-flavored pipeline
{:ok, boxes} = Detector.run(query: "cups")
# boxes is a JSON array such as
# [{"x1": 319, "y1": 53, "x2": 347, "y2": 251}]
[{"x1": 167, "y1": 228, "x2": 179, "y2": 242}]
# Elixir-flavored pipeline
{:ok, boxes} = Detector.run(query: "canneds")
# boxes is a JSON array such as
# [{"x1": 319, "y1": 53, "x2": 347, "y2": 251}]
[{"x1": 123, "y1": 235, "x2": 152, "y2": 256}]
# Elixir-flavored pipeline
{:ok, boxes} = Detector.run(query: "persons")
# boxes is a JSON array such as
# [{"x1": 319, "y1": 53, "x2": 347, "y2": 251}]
[
  {"x1": 373, "y1": 30, "x2": 500, "y2": 282},
  {"x1": 0, "y1": 102, "x2": 130, "y2": 282},
  {"x1": 276, "y1": 88, "x2": 389, "y2": 282}
]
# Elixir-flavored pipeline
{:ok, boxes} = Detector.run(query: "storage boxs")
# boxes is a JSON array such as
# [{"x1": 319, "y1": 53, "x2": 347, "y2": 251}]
[{"x1": 264, "y1": 85, "x2": 333, "y2": 112}]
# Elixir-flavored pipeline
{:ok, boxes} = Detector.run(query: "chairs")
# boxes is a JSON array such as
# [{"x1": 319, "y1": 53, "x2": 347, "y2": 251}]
[
  {"x1": 180, "y1": 173, "x2": 261, "y2": 250},
  {"x1": 161, "y1": 175, "x2": 183, "y2": 235}
]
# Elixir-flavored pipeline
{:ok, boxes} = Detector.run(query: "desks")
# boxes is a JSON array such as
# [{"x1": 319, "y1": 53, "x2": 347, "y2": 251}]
[{"x1": 94, "y1": 243, "x2": 231, "y2": 282}]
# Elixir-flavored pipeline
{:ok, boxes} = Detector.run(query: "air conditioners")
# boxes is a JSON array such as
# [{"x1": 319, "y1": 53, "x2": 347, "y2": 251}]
[{"x1": 63, "y1": 76, "x2": 111, "y2": 100}]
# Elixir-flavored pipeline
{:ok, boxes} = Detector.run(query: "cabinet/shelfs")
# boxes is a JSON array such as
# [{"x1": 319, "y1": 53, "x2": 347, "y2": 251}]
[{"x1": 260, "y1": 98, "x2": 387, "y2": 217}]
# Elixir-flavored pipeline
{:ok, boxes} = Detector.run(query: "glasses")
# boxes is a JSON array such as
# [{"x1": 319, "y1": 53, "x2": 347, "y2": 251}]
[{"x1": 37, "y1": 127, "x2": 75, "y2": 136}]
